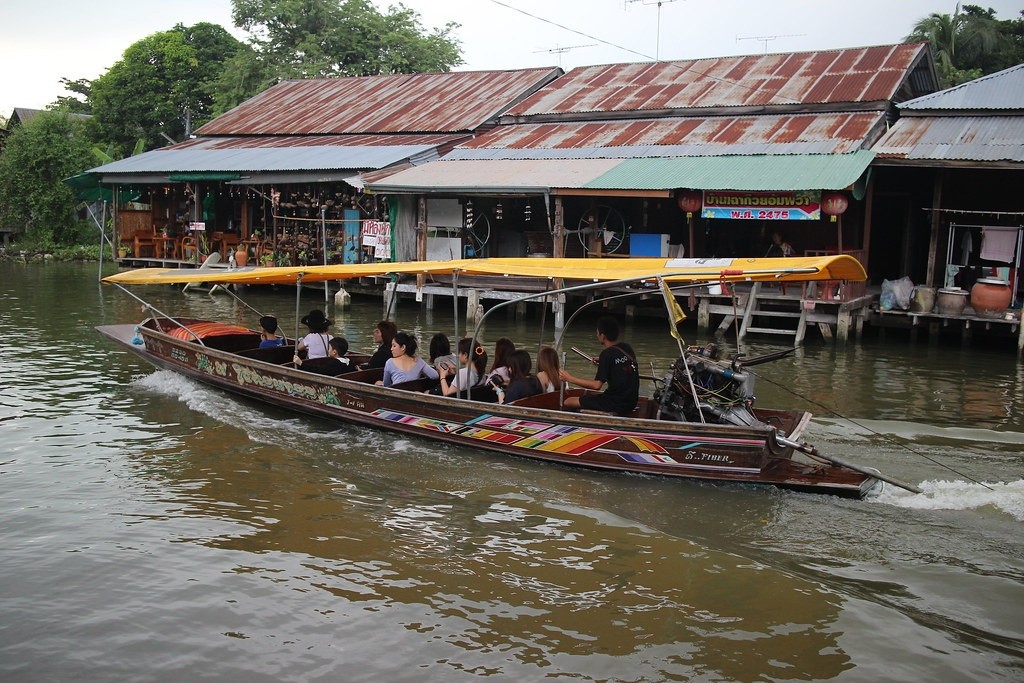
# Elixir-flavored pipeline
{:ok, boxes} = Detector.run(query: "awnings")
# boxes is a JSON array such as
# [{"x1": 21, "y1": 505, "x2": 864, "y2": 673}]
[{"x1": 62, "y1": 137, "x2": 879, "y2": 207}]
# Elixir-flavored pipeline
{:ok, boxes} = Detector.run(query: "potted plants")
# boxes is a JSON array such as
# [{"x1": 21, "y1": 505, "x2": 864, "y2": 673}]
[
  {"x1": 197, "y1": 232, "x2": 212, "y2": 265},
  {"x1": 117, "y1": 244, "x2": 130, "y2": 259},
  {"x1": 235, "y1": 244, "x2": 248, "y2": 269}
]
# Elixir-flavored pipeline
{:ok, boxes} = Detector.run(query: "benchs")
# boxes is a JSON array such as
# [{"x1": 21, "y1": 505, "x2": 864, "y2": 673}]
[
  {"x1": 181, "y1": 236, "x2": 212, "y2": 262},
  {"x1": 134, "y1": 230, "x2": 170, "y2": 258},
  {"x1": 217, "y1": 232, "x2": 242, "y2": 263}
]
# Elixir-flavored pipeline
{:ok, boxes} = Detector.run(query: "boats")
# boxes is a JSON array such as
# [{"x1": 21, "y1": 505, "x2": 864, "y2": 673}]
[{"x1": 94, "y1": 255, "x2": 925, "y2": 500}]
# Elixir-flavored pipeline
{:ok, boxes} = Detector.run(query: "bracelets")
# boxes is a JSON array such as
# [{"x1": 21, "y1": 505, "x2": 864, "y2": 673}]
[
  {"x1": 496, "y1": 386, "x2": 504, "y2": 395},
  {"x1": 440, "y1": 377, "x2": 446, "y2": 381}
]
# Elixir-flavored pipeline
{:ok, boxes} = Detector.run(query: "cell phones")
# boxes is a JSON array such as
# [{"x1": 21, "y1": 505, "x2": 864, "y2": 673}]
[
  {"x1": 298, "y1": 337, "x2": 303, "y2": 342},
  {"x1": 441, "y1": 363, "x2": 448, "y2": 370}
]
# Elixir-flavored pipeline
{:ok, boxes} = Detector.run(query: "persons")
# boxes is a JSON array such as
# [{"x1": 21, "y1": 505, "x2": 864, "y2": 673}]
[
  {"x1": 355, "y1": 319, "x2": 397, "y2": 371},
  {"x1": 438, "y1": 337, "x2": 488, "y2": 398},
  {"x1": 426, "y1": 332, "x2": 464, "y2": 379},
  {"x1": 480, "y1": 336, "x2": 516, "y2": 387},
  {"x1": 562, "y1": 316, "x2": 640, "y2": 415},
  {"x1": 490, "y1": 349, "x2": 544, "y2": 405},
  {"x1": 535, "y1": 347, "x2": 569, "y2": 393},
  {"x1": 293, "y1": 337, "x2": 359, "y2": 376},
  {"x1": 258, "y1": 313, "x2": 285, "y2": 348},
  {"x1": 297, "y1": 308, "x2": 337, "y2": 361},
  {"x1": 374, "y1": 332, "x2": 439, "y2": 386},
  {"x1": 767, "y1": 232, "x2": 797, "y2": 257}
]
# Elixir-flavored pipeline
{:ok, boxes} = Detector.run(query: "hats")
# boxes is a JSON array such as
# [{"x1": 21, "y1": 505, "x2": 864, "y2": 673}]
[
  {"x1": 301, "y1": 310, "x2": 334, "y2": 327},
  {"x1": 260, "y1": 317, "x2": 278, "y2": 328}
]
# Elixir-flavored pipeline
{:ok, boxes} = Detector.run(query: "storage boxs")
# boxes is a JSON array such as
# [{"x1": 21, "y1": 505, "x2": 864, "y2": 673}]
[
  {"x1": 937, "y1": 286, "x2": 969, "y2": 316},
  {"x1": 970, "y1": 276, "x2": 1012, "y2": 321},
  {"x1": 912, "y1": 284, "x2": 937, "y2": 314}
]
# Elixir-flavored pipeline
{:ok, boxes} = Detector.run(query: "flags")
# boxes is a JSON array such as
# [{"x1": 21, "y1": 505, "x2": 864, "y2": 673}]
[{"x1": 658, "y1": 274, "x2": 689, "y2": 346}]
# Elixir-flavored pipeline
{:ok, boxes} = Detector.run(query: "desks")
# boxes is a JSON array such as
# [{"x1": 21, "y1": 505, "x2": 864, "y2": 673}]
[{"x1": 151, "y1": 235, "x2": 180, "y2": 261}]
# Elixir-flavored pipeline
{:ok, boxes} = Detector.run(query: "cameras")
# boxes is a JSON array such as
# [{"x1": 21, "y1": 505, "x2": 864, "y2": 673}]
[{"x1": 487, "y1": 374, "x2": 504, "y2": 389}]
